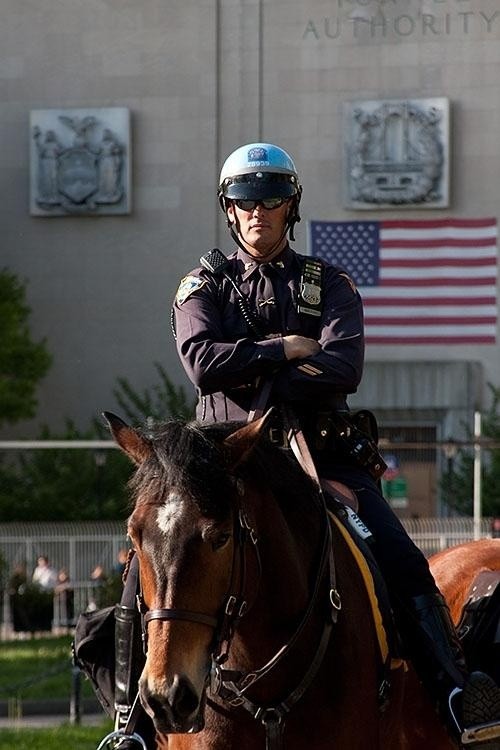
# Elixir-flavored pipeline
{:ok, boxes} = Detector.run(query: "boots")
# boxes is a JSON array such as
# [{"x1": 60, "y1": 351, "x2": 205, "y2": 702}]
[
  {"x1": 393, "y1": 592, "x2": 500, "y2": 750},
  {"x1": 112, "y1": 603, "x2": 153, "y2": 750}
]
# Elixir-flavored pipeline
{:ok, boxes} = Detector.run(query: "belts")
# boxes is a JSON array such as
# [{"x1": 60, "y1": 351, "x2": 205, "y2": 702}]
[{"x1": 259, "y1": 413, "x2": 355, "y2": 449}]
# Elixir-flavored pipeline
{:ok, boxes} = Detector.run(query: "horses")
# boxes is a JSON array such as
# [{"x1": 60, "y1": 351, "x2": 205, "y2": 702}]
[{"x1": 99, "y1": 402, "x2": 457, "y2": 749}]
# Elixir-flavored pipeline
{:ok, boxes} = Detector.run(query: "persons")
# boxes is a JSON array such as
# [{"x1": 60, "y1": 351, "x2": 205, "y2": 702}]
[
  {"x1": 407, "y1": 108, "x2": 444, "y2": 162},
  {"x1": 31, "y1": 123, "x2": 67, "y2": 204},
  {"x1": 353, "y1": 109, "x2": 373, "y2": 161},
  {"x1": 106, "y1": 142, "x2": 500, "y2": 750},
  {"x1": 82, "y1": 128, "x2": 124, "y2": 203},
  {"x1": 0, "y1": 547, "x2": 132, "y2": 641}
]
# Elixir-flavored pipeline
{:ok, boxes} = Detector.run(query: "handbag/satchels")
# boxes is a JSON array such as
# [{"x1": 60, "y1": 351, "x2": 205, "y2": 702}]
[
  {"x1": 74, "y1": 605, "x2": 116, "y2": 721},
  {"x1": 454, "y1": 569, "x2": 500, "y2": 646}
]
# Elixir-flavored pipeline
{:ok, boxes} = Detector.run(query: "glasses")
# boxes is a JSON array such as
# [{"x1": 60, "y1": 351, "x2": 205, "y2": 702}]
[{"x1": 232, "y1": 197, "x2": 289, "y2": 211}]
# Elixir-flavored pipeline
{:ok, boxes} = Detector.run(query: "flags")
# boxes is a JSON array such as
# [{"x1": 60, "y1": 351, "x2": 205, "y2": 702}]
[{"x1": 303, "y1": 215, "x2": 500, "y2": 348}]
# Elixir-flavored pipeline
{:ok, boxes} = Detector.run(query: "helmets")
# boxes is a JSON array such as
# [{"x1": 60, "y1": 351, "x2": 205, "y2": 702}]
[{"x1": 217, "y1": 143, "x2": 301, "y2": 200}]
